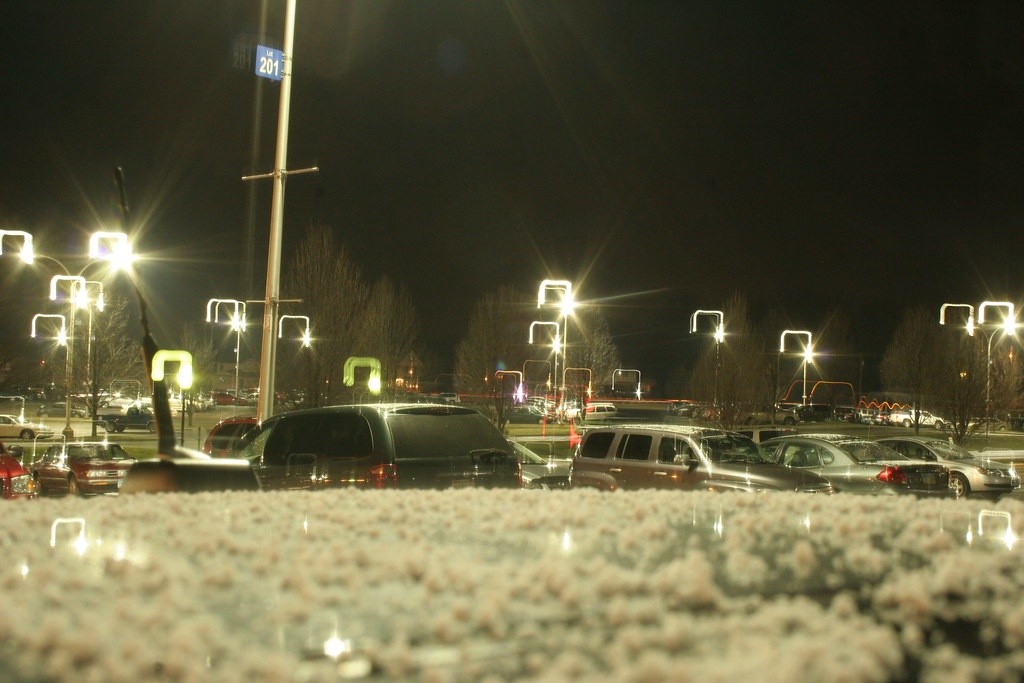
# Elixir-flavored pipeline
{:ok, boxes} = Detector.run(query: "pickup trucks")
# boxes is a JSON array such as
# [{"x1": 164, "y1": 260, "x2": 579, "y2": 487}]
[{"x1": 891, "y1": 410, "x2": 946, "y2": 430}]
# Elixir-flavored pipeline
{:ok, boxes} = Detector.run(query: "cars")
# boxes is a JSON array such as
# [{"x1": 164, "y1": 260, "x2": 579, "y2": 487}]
[
  {"x1": 38, "y1": 388, "x2": 259, "y2": 433},
  {"x1": 568, "y1": 424, "x2": 834, "y2": 494},
  {"x1": 118, "y1": 403, "x2": 522, "y2": 494},
  {"x1": 493, "y1": 395, "x2": 617, "y2": 423},
  {"x1": 861, "y1": 414, "x2": 891, "y2": 426},
  {"x1": 672, "y1": 402, "x2": 800, "y2": 426},
  {"x1": 796, "y1": 403, "x2": 831, "y2": 422},
  {"x1": 0, "y1": 414, "x2": 138, "y2": 499},
  {"x1": 851, "y1": 437, "x2": 1021, "y2": 502},
  {"x1": 507, "y1": 439, "x2": 569, "y2": 488},
  {"x1": 950, "y1": 417, "x2": 979, "y2": 429},
  {"x1": 966, "y1": 417, "x2": 1011, "y2": 433},
  {"x1": 755, "y1": 433, "x2": 956, "y2": 501},
  {"x1": 833, "y1": 407, "x2": 875, "y2": 423}
]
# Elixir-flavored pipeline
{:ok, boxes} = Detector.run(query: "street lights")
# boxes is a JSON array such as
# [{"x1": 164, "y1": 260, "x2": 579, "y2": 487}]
[
  {"x1": 690, "y1": 310, "x2": 724, "y2": 423},
  {"x1": 0, "y1": 230, "x2": 130, "y2": 440},
  {"x1": 940, "y1": 303, "x2": 1014, "y2": 438},
  {"x1": 529, "y1": 280, "x2": 572, "y2": 424},
  {"x1": 780, "y1": 330, "x2": 812, "y2": 405}
]
